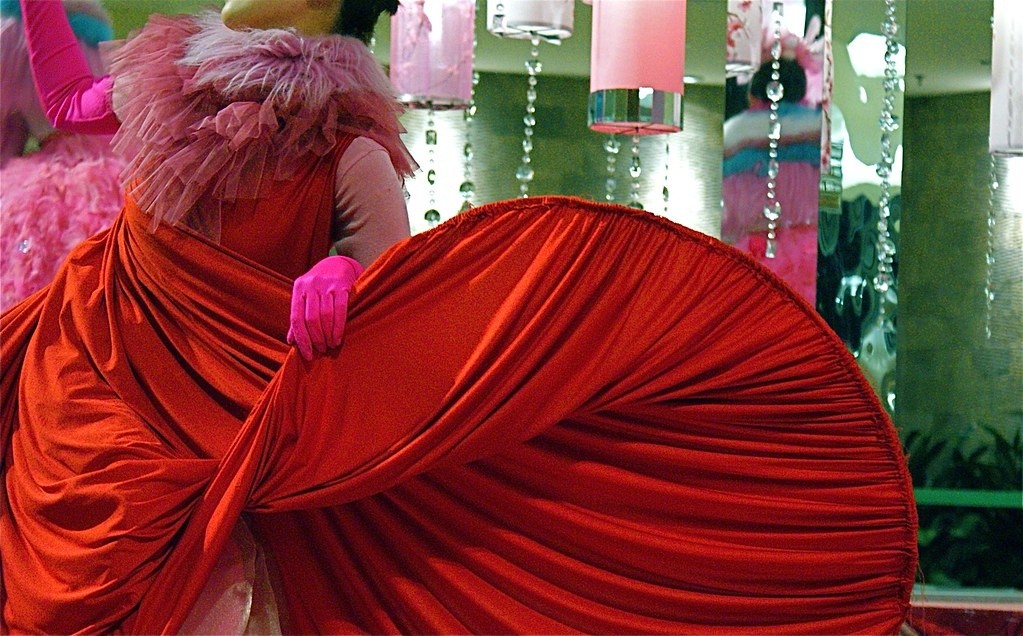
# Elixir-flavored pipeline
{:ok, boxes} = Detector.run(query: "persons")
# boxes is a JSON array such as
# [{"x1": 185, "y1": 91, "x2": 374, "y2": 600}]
[
  {"x1": 398, "y1": 1, "x2": 432, "y2": 96},
  {"x1": 0, "y1": 0, "x2": 413, "y2": 636},
  {"x1": 722, "y1": 58, "x2": 824, "y2": 311}
]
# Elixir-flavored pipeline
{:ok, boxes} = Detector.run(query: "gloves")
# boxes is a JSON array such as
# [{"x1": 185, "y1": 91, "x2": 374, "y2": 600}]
[{"x1": 286, "y1": 256, "x2": 363, "y2": 359}]
